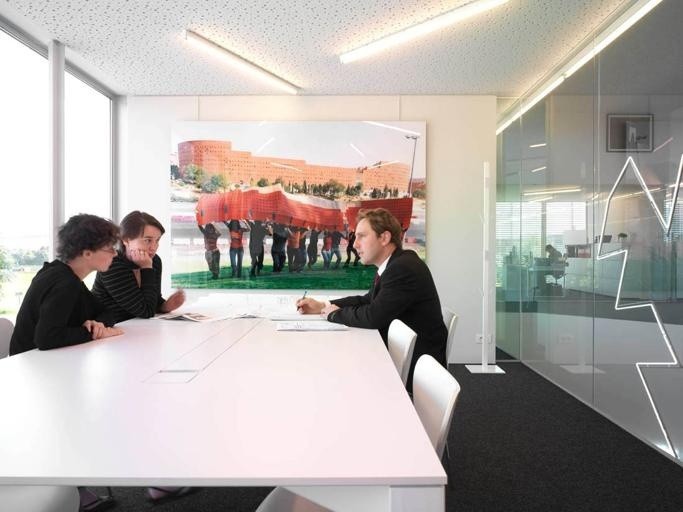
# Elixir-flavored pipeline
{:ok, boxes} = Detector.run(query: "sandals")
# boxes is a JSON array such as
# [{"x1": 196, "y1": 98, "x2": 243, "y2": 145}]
[
  {"x1": 146, "y1": 487, "x2": 202, "y2": 504},
  {"x1": 79, "y1": 495, "x2": 114, "y2": 512}
]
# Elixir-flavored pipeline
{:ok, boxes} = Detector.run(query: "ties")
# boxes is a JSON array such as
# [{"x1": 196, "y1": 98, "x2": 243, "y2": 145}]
[{"x1": 374, "y1": 273, "x2": 379, "y2": 286}]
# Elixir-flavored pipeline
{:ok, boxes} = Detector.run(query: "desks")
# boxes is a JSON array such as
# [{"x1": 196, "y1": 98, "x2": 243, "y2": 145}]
[{"x1": 0, "y1": 304, "x2": 449, "y2": 512}]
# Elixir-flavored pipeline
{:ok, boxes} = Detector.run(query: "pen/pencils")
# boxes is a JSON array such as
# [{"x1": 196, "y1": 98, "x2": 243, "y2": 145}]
[{"x1": 296, "y1": 291, "x2": 308, "y2": 311}]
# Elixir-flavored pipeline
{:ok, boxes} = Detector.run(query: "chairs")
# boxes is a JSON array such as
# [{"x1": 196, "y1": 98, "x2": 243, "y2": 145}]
[
  {"x1": 544, "y1": 253, "x2": 568, "y2": 295},
  {"x1": 386, "y1": 303, "x2": 459, "y2": 464},
  {"x1": 1, "y1": 318, "x2": 82, "y2": 512}
]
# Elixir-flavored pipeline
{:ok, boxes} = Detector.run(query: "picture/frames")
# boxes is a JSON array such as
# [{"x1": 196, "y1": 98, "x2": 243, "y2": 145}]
[{"x1": 606, "y1": 112, "x2": 655, "y2": 154}]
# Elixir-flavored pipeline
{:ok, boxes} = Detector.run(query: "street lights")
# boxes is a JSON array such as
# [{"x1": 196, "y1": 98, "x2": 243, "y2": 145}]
[{"x1": 405, "y1": 134, "x2": 420, "y2": 198}]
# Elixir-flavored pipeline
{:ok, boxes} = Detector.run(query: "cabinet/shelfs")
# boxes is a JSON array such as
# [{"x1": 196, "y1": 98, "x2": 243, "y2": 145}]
[{"x1": 502, "y1": 261, "x2": 566, "y2": 303}]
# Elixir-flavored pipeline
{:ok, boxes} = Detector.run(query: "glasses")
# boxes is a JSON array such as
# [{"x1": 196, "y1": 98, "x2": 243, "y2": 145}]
[{"x1": 98, "y1": 245, "x2": 116, "y2": 254}]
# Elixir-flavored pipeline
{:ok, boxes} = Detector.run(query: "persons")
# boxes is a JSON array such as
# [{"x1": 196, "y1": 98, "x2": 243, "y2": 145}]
[
  {"x1": 89, "y1": 210, "x2": 187, "y2": 323},
  {"x1": 294, "y1": 206, "x2": 448, "y2": 402},
  {"x1": 544, "y1": 243, "x2": 565, "y2": 277},
  {"x1": 8, "y1": 211, "x2": 125, "y2": 355},
  {"x1": 197, "y1": 220, "x2": 361, "y2": 280}
]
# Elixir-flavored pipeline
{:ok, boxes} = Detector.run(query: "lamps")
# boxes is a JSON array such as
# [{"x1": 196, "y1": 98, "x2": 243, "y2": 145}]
[
  {"x1": 179, "y1": 28, "x2": 303, "y2": 96},
  {"x1": 338, "y1": 0, "x2": 512, "y2": 64}
]
